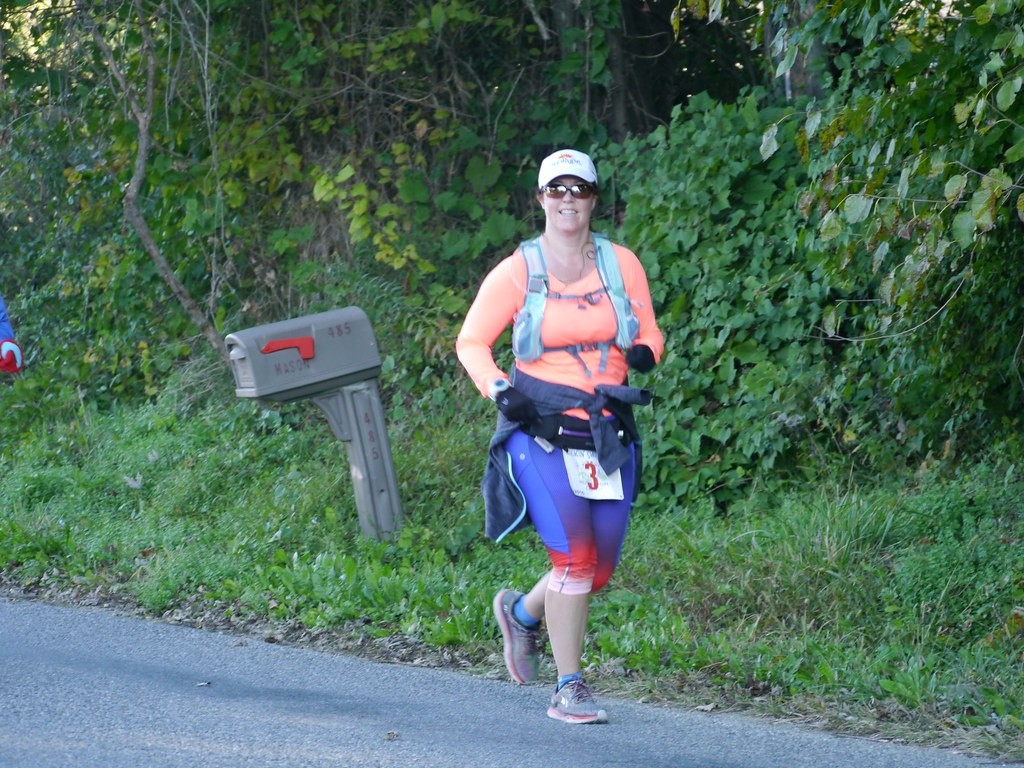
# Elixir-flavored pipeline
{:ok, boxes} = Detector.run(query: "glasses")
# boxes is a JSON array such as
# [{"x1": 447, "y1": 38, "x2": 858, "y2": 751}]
[{"x1": 540, "y1": 183, "x2": 596, "y2": 199}]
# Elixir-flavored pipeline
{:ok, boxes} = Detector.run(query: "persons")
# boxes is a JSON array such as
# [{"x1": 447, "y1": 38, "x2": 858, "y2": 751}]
[
  {"x1": 454, "y1": 150, "x2": 666, "y2": 725},
  {"x1": 0, "y1": 293, "x2": 23, "y2": 374}
]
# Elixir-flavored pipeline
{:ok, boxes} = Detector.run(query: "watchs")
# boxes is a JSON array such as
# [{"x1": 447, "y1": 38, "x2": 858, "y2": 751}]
[{"x1": 488, "y1": 378, "x2": 513, "y2": 402}]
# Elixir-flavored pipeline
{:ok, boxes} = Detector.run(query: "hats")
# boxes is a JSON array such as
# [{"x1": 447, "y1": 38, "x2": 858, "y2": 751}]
[{"x1": 538, "y1": 149, "x2": 597, "y2": 190}]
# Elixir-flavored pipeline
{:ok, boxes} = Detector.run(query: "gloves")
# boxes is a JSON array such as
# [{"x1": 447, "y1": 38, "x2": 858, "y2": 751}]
[
  {"x1": 496, "y1": 387, "x2": 544, "y2": 426},
  {"x1": 626, "y1": 345, "x2": 656, "y2": 373},
  {"x1": 0, "y1": 339, "x2": 23, "y2": 373}
]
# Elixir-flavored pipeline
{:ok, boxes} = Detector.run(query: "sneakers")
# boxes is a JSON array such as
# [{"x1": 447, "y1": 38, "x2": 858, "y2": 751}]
[
  {"x1": 493, "y1": 589, "x2": 539, "y2": 684},
  {"x1": 547, "y1": 676, "x2": 608, "y2": 724}
]
